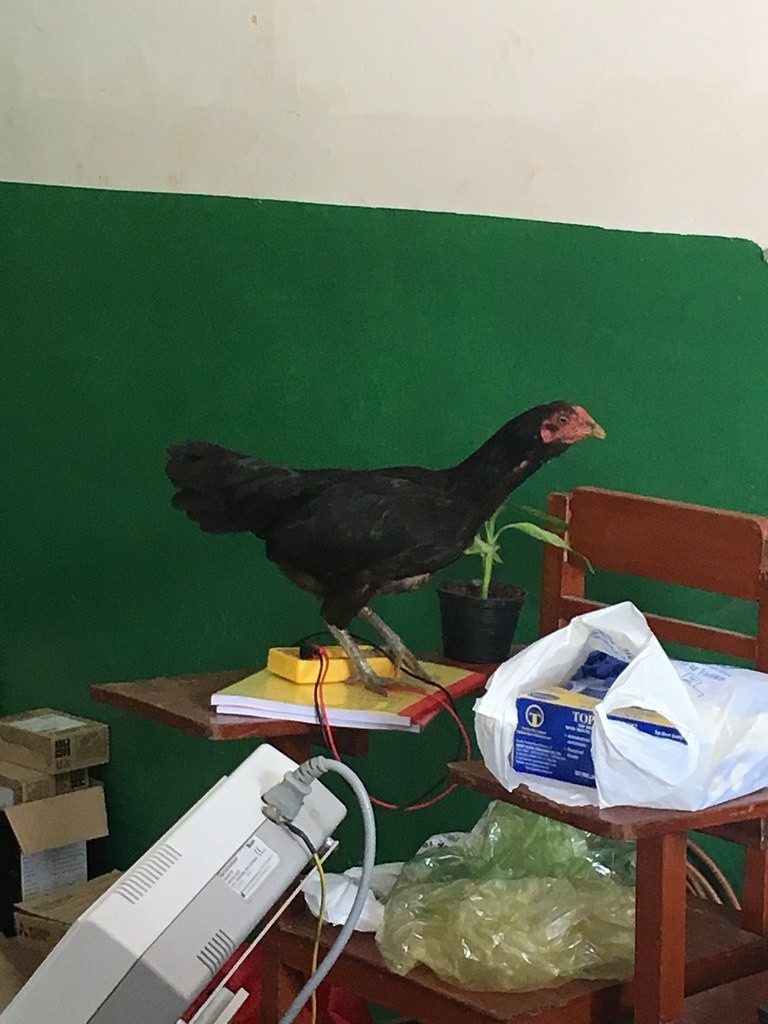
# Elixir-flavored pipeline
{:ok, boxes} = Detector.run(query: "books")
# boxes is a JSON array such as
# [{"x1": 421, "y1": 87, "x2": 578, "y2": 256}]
[{"x1": 210, "y1": 660, "x2": 486, "y2": 733}]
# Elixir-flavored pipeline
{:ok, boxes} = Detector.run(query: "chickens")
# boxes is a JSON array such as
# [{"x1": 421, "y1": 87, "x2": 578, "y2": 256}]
[{"x1": 166, "y1": 401, "x2": 606, "y2": 696}]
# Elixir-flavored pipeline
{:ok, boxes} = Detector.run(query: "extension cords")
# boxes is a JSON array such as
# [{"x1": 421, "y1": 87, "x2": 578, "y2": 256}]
[{"x1": 269, "y1": 645, "x2": 399, "y2": 685}]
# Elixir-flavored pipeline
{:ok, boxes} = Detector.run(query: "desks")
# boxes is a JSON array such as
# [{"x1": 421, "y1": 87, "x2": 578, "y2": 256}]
[{"x1": 106, "y1": 650, "x2": 504, "y2": 782}]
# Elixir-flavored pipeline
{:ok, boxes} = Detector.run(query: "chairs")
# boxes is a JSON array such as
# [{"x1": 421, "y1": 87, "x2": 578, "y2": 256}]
[{"x1": 257, "y1": 483, "x2": 768, "y2": 1024}]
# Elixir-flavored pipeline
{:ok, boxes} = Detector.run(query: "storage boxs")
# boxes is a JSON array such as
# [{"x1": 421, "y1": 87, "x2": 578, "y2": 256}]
[{"x1": 0, "y1": 708, "x2": 135, "y2": 1018}]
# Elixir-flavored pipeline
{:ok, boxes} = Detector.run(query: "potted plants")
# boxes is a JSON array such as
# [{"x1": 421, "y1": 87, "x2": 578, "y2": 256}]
[{"x1": 437, "y1": 490, "x2": 597, "y2": 666}]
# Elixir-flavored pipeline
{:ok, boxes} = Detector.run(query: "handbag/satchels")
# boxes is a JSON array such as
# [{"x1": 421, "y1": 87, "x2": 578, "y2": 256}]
[{"x1": 474, "y1": 599, "x2": 768, "y2": 814}]
